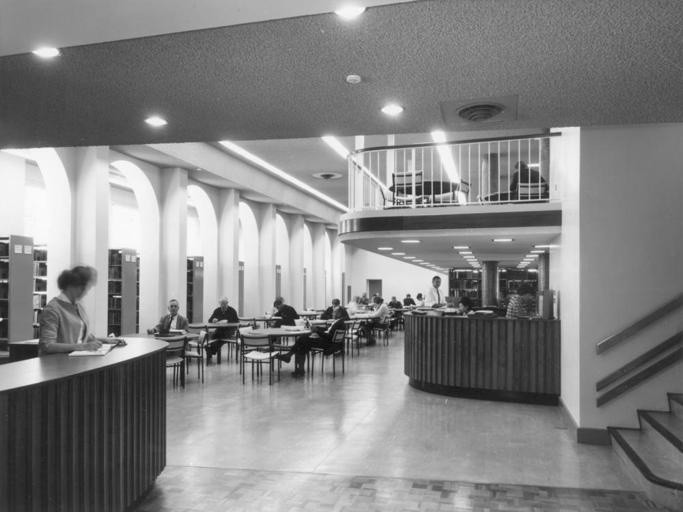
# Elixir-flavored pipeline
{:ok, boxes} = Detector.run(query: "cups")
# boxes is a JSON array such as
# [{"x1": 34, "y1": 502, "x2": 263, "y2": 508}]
[{"x1": 264, "y1": 314, "x2": 272, "y2": 320}]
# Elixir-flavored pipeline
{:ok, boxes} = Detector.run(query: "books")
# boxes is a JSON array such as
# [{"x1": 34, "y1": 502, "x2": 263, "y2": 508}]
[{"x1": 68, "y1": 343, "x2": 116, "y2": 356}]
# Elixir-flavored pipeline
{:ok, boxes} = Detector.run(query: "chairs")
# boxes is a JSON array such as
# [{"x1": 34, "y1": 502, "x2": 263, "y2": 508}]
[
  {"x1": 152, "y1": 307, "x2": 409, "y2": 386},
  {"x1": 377, "y1": 170, "x2": 547, "y2": 207}
]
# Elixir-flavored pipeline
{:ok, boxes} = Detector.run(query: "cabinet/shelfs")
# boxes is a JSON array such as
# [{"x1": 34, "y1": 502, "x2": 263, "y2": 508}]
[{"x1": 447, "y1": 265, "x2": 539, "y2": 309}]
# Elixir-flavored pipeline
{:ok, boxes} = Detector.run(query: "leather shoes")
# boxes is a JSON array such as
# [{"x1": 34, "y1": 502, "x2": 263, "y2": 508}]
[
  {"x1": 275, "y1": 354, "x2": 290, "y2": 364},
  {"x1": 291, "y1": 370, "x2": 305, "y2": 376}
]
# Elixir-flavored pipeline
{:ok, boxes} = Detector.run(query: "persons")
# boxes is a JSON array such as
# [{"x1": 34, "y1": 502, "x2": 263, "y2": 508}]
[
  {"x1": 349, "y1": 292, "x2": 425, "y2": 346},
  {"x1": 205, "y1": 299, "x2": 239, "y2": 358},
  {"x1": 505, "y1": 283, "x2": 536, "y2": 318},
  {"x1": 510, "y1": 161, "x2": 548, "y2": 192},
  {"x1": 457, "y1": 295, "x2": 476, "y2": 316},
  {"x1": 424, "y1": 276, "x2": 447, "y2": 308},
  {"x1": 146, "y1": 299, "x2": 189, "y2": 336},
  {"x1": 271, "y1": 297, "x2": 299, "y2": 329},
  {"x1": 275, "y1": 305, "x2": 346, "y2": 376},
  {"x1": 37, "y1": 265, "x2": 126, "y2": 356}
]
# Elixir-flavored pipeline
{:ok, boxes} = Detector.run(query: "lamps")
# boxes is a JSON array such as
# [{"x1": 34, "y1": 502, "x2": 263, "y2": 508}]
[
  {"x1": 433, "y1": 131, "x2": 466, "y2": 203},
  {"x1": 324, "y1": 137, "x2": 352, "y2": 158}
]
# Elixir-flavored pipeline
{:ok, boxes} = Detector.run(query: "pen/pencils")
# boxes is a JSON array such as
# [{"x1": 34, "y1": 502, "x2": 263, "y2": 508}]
[{"x1": 90, "y1": 333, "x2": 96, "y2": 341}]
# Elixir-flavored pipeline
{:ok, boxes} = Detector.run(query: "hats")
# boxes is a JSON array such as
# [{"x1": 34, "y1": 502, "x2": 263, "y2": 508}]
[{"x1": 458, "y1": 296, "x2": 473, "y2": 305}]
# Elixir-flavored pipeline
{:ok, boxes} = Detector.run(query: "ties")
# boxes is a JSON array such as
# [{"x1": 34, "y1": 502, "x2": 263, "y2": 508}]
[
  {"x1": 169, "y1": 316, "x2": 174, "y2": 329},
  {"x1": 436, "y1": 289, "x2": 441, "y2": 305}
]
